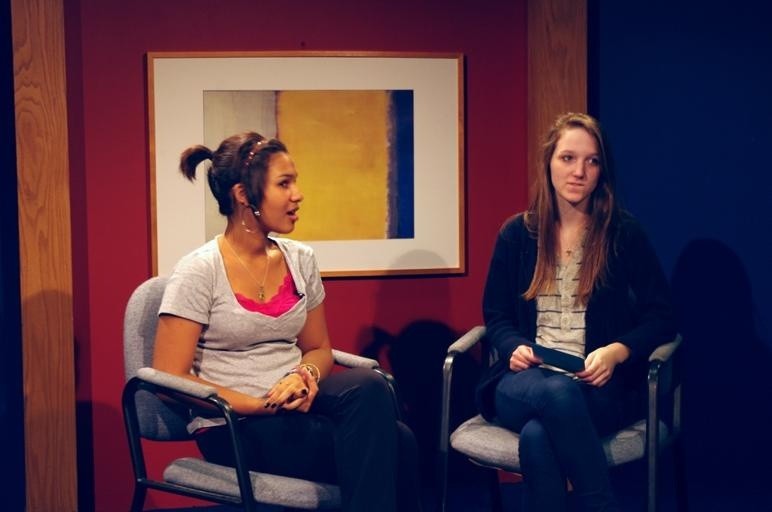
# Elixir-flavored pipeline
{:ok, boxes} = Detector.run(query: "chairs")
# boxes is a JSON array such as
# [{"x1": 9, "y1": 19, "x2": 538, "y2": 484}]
[
  {"x1": 121, "y1": 274, "x2": 395, "y2": 512},
  {"x1": 434, "y1": 295, "x2": 685, "y2": 511}
]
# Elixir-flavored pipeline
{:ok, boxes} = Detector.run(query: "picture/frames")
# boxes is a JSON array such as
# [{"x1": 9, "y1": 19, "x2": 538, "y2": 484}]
[{"x1": 144, "y1": 48, "x2": 466, "y2": 282}]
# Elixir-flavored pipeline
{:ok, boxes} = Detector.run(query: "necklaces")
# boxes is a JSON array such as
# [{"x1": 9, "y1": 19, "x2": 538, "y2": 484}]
[{"x1": 223, "y1": 232, "x2": 270, "y2": 301}]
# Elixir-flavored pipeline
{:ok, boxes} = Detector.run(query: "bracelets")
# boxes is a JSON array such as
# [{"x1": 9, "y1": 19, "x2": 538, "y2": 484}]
[{"x1": 284, "y1": 363, "x2": 320, "y2": 384}]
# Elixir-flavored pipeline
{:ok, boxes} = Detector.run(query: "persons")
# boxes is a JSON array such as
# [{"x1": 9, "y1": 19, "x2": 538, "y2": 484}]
[
  {"x1": 475, "y1": 112, "x2": 672, "y2": 512},
  {"x1": 151, "y1": 131, "x2": 422, "y2": 512}
]
